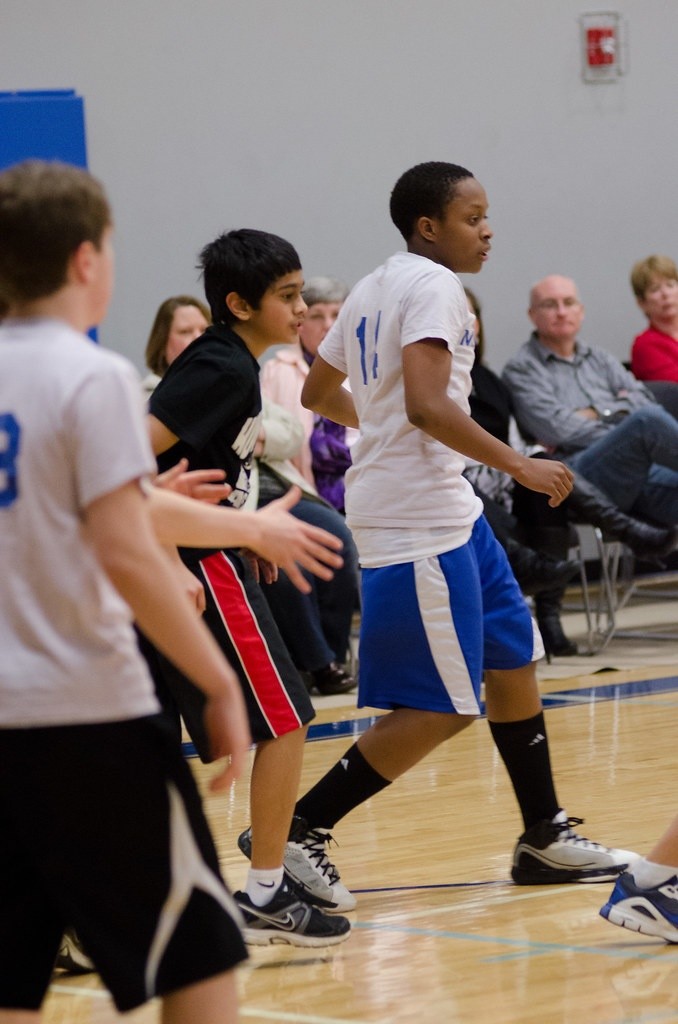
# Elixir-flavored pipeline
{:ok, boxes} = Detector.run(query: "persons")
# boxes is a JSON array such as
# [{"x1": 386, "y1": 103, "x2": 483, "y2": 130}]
[
  {"x1": 140, "y1": 278, "x2": 368, "y2": 692},
  {"x1": 599, "y1": 812, "x2": 678, "y2": 944},
  {"x1": 234, "y1": 161, "x2": 642, "y2": 912},
  {"x1": 463, "y1": 286, "x2": 678, "y2": 666},
  {"x1": 0, "y1": 158, "x2": 252, "y2": 1023},
  {"x1": 502, "y1": 275, "x2": 678, "y2": 528},
  {"x1": 56, "y1": 228, "x2": 354, "y2": 972},
  {"x1": 630, "y1": 256, "x2": 678, "y2": 385}
]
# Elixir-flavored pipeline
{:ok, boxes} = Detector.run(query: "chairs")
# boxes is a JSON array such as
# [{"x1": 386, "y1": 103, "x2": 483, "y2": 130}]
[{"x1": 523, "y1": 448, "x2": 677, "y2": 656}]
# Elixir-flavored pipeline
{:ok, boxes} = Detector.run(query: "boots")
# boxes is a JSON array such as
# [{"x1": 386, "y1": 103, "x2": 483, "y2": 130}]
[
  {"x1": 533, "y1": 524, "x2": 578, "y2": 664},
  {"x1": 551, "y1": 461, "x2": 678, "y2": 568}
]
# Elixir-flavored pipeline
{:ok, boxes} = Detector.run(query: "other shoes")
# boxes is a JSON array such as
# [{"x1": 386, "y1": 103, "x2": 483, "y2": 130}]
[{"x1": 311, "y1": 660, "x2": 358, "y2": 695}]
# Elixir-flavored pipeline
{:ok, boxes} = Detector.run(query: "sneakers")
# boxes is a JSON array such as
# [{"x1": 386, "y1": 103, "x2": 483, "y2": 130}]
[
  {"x1": 239, "y1": 827, "x2": 357, "y2": 913},
  {"x1": 512, "y1": 807, "x2": 642, "y2": 882},
  {"x1": 233, "y1": 871, "x2": 352, "y2": 947},
  {"x1": 53, "y1": 928, "x2": 95, "y2": 971},
  {"x1": 599, "y1": 872, "x2": 678, "y2": 942}
]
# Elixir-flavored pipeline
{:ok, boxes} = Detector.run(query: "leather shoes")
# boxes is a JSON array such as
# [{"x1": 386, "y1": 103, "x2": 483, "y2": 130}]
[{"x1": 500, "y1": 535, "x2": 580, "y2": 593}]
